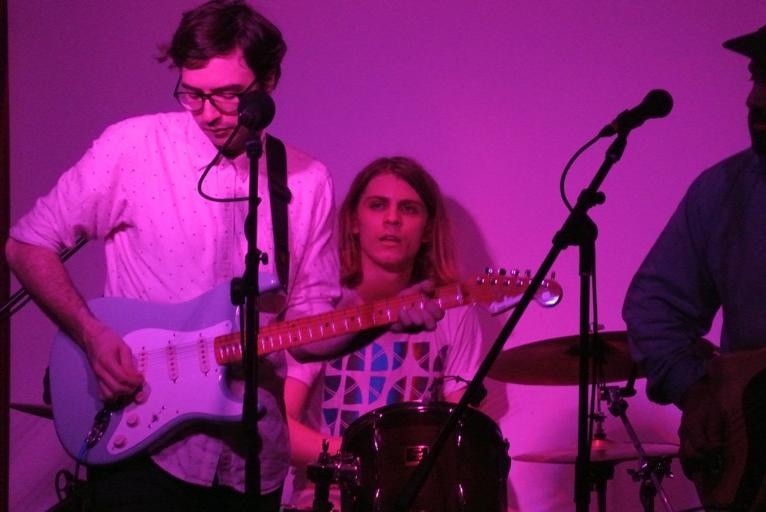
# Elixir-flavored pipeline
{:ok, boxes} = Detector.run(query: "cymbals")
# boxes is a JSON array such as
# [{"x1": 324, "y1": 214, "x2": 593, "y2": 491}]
[
  {"x1": 7, "y1": 401, "x2": 50, "y2": 419},
  {"x1": 511, "y1": 438, "x2": 681, "y2": 466},
  {"x1": 487, "y1": 329, "x2": 718, "y2": 385}
]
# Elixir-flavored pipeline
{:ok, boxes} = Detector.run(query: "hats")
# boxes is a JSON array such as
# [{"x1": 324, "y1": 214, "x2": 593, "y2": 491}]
[{"x1": 723, "y1": 25, "x2": 766, "y2": 66}]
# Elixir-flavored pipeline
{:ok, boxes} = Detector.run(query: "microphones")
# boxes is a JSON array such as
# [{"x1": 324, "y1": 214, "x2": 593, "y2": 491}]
[
  {"x1": 598, "y1": 88, "x2": 674, "y2": 137},
  {"x1": 236, "y1": 90, "x2": 276, "y2": 132}
]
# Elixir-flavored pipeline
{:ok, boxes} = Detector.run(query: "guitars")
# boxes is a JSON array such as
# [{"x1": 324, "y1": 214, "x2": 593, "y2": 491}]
[
  {"x1": 681, "y1": 349, "x2": 766, "y2": 511},
  {"x1": 49, "y1": 266, "x2": 562, "y2": 468}
]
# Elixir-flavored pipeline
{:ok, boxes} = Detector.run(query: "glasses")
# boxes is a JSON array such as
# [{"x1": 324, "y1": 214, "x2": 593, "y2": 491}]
[{"x1": 174, "y1": 75, "x2": 259, "y2": 114}]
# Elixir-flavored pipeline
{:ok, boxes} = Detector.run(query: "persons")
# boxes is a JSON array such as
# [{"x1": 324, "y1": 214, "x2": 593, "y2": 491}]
[
  {"x1": 621, "y1": 27, "x2": 763, "y2": 511},
  {"x1": 3, "y1": 0, "x2": 445, "y2": 511},
  {"x1": 279, "y1": 155, "x2": 485, "y2": 510}
]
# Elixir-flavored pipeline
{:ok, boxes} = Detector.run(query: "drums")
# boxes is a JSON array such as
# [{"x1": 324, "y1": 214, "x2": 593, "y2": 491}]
[
  {"x1": 285, "y1": 484, "x2": 341, "y2": 512},
  {"x1": 336, "y1": 400, "x2": 511, "y2": 511}
]
